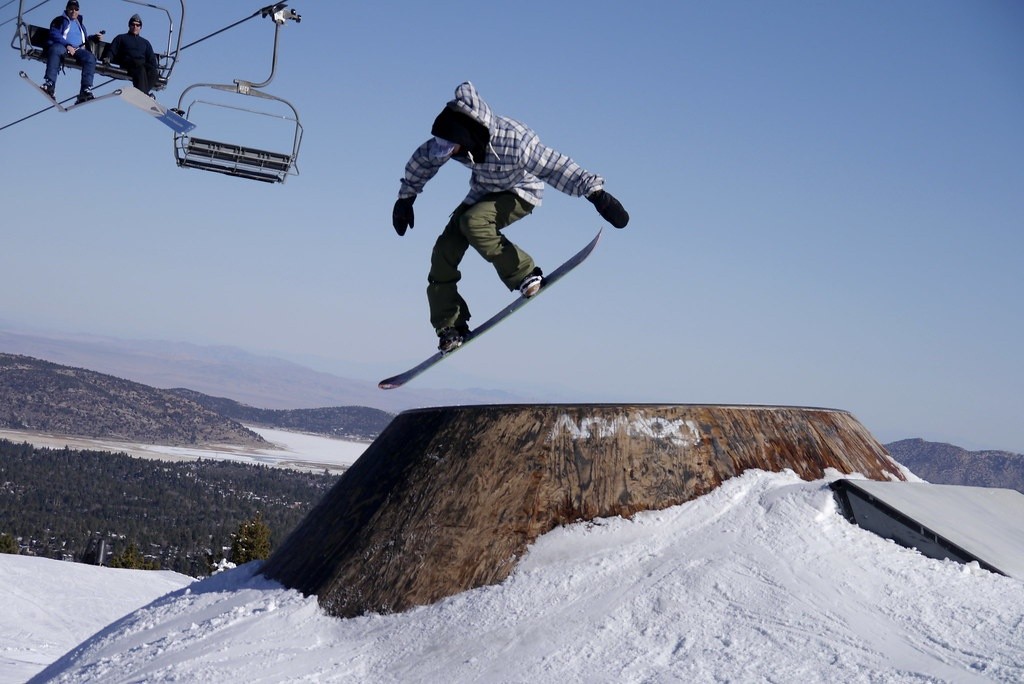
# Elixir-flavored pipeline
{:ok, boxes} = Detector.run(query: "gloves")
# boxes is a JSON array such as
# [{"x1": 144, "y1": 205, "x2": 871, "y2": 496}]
[
  {"x1": 102, "y1": 57, "x2": 111, "y2": 67},
  {"x1": 393, "y1": 193, "x2": 414, "y2": 236},
  {"x1": 588, "y1": 189, "x2": 629, "y2": 228}
]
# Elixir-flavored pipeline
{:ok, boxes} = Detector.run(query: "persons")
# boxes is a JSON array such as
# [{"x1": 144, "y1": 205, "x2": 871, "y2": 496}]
[
  {"x1": 103, "y1": 13, "x2": 160, "y2": 99},
  {"x1": 39, "y1": 0, "x2": 102, "y2": 105},
  {"x1": 392, "y1": 81, "x2": 628, "y2": 353}
]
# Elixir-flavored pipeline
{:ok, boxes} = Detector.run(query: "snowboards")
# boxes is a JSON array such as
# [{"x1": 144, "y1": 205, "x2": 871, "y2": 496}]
[
  {"x1": 120, "y1": 84, "x2": 198, "y2": 135},
  {"x1": 377, "y1": 227, "x2": 603, "y2": 390}
]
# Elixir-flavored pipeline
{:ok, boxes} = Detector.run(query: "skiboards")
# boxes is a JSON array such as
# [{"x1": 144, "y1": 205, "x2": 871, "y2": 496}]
[{"x1": 18, "y1": 70, "x2": 122, "y2": 113}]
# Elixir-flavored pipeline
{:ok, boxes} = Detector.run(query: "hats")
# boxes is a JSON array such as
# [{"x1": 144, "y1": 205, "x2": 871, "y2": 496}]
[
  {"x1": 429, "y1": 135, "x2": 455, "y2": 157},
  {"x1": 68, "y1": 0, "x2": 79, "y2": 7},
  {"x1": 128, "y1": 13, "x2": 142, "y2": 28}
]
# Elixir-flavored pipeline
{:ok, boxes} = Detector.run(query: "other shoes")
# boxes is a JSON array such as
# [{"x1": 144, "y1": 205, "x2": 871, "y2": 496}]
[
  {"x1": 76, "y1": 86, "x2": 93, "y2": 102},
  {"x1": 439, "y1": 319, "x2": 471, "y2": 351},
  {"x1": 520, "y1": 269, "x2": 544, "y2": 297},
  {"x1": 39, "y1": 77, "x2": 54, "y2": 95}
]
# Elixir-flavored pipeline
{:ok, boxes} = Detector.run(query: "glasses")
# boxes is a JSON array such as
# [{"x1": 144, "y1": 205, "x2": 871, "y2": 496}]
[
  {"x1": 131, "y1": 22, "x2": 141, "y2": 28},
  {"x1": 67, "y1": 6, "x2": 79, "y2": 11}
]
